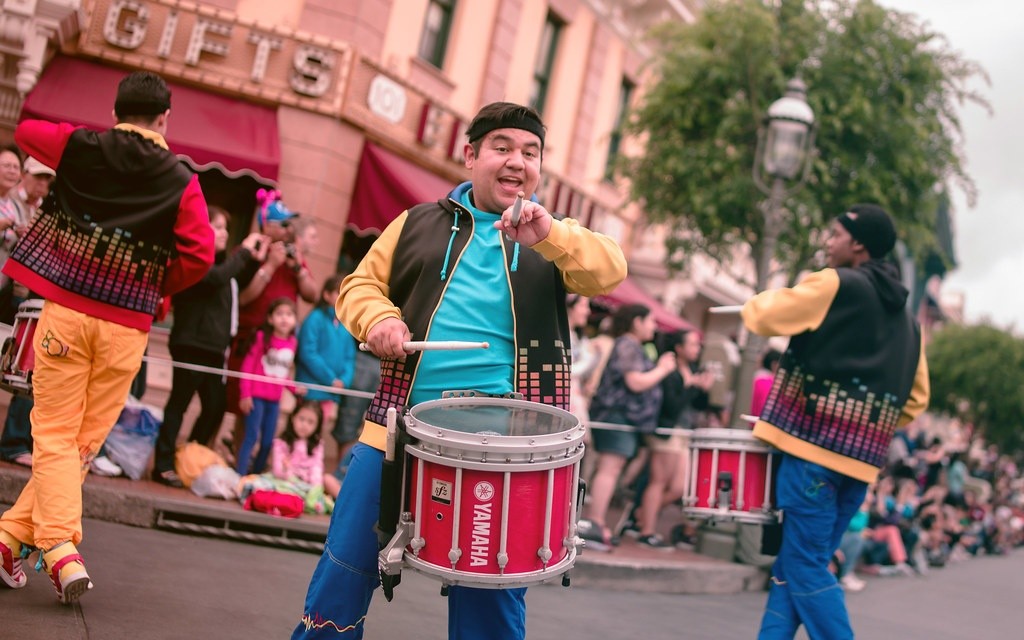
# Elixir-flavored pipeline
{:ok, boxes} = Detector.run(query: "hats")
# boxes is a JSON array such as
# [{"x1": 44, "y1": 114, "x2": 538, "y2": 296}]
[
  {"x1": 839, "y1": 206, "x2": 897, "y2": 259},
  {"x1": 24, "y1": 155, "x2": 57, "y2": 178}
]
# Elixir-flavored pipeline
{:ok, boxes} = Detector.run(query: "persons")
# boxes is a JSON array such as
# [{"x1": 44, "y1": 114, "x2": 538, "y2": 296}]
[
  {"x1": 0, "y1": 69, "x2": 213, "y2": 607},
  {"x1": 743, "y1": 204, "x2": 931, "y2": 640},
  {"x1": 0, "y1": 148, "x2": 781, "y2": 550},
  {"x1": 287, "y1": 102, "x2": 628, "y2": 640},
  {"x1": 825, "y1": 421, "x2": 1024, "y2": 592}
]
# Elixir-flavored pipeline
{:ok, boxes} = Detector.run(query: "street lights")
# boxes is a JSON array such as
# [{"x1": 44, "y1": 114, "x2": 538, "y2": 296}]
[{"x1": 729, "y1": 62, "x2": 819, "y2": 431}]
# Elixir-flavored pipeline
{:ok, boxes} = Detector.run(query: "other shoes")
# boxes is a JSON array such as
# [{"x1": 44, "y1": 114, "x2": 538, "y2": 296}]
[
  {"x1": 151, "y1": 469, "x2": 184, "y2": 489},
  {"x1": 840, "y1": 572, "x2": 866, "y2": 593},
  {"x1": 576, "y1": 503, "x2": 699, "y2": 552}
]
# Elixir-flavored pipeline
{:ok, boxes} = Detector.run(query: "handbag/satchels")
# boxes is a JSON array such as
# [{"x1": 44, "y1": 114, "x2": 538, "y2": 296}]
[
  {"x1": 103, "y1": 393, "x2": 165, "y2": 480},
  {"x1": 175, "y1": 440, "x2": 304, "y2": 519}
]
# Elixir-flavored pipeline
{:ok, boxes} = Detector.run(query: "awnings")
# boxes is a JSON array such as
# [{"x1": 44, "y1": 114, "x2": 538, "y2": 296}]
[
  {"x1": 18, "y1": 54, "x2": 284, "y2": 190},
  {"x1": 593, "y1": 277, "x2": 709, "y2": 342},
  {"x1": 347, "y1": 146, "x2": 461, "y2": 237}
]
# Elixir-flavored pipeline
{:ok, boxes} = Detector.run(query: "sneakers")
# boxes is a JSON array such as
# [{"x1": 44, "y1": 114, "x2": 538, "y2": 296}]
[
  {"x1": 40, "y1": 543, "x2": 93, "y2": 604},
  {"x1": 1, "y1": 540, "x2": 27, "y2": 588}
]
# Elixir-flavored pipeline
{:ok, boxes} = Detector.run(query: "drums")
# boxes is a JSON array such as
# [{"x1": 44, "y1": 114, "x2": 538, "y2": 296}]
[
  {"x1": 682, "y1": 427, "x2": 782, "y2": 525},
  {"x1": 0, "y1": 299, "x2": 46, "y2": 401},
  {"x1": 402, "y1": 397, "x2": 586, "y2": 588}
]
